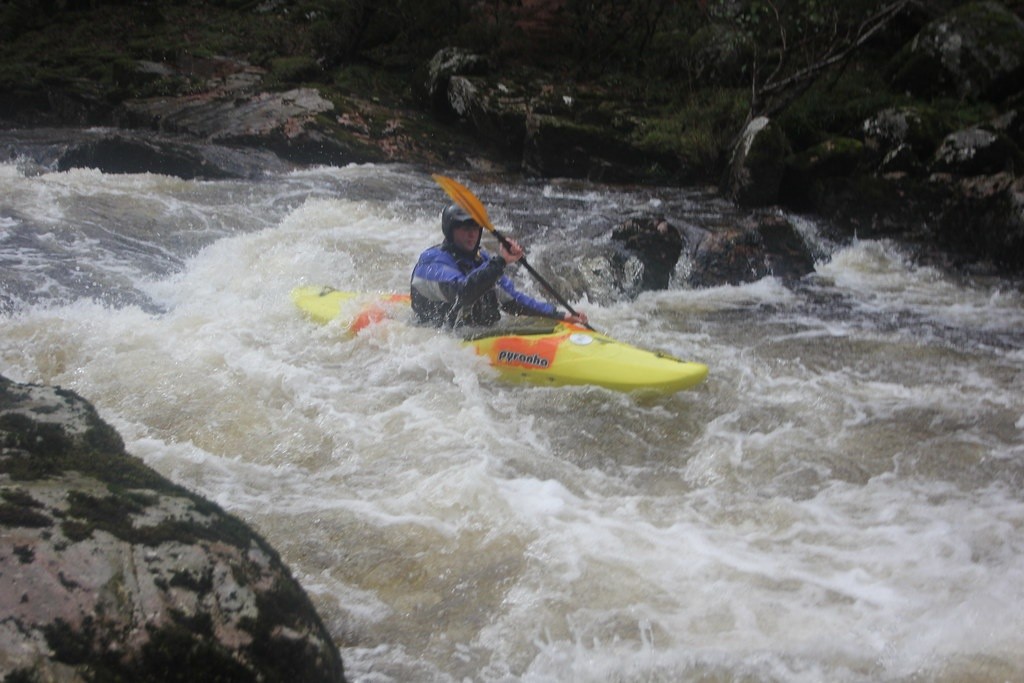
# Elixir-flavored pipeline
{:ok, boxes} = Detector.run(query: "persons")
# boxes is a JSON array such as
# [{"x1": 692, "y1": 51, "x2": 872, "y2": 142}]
[{"x1": 409, "y1": 197, "x2": 589, "y2": 334}]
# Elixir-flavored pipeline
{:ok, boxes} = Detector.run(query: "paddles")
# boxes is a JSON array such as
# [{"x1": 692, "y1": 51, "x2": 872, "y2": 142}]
[{"x1": 431, "y1": 172, "x2": 596, "y2": 333}]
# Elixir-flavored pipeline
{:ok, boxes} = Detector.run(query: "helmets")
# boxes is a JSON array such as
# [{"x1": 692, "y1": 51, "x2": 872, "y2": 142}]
[{"x1": 442, "y1": 204, "x2": 483, "y2": 240}]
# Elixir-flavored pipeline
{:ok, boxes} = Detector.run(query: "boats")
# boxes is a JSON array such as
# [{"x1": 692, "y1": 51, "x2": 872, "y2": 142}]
[{"x1": 292, "y1": 278, "x2": 712, "y2": 401}]
